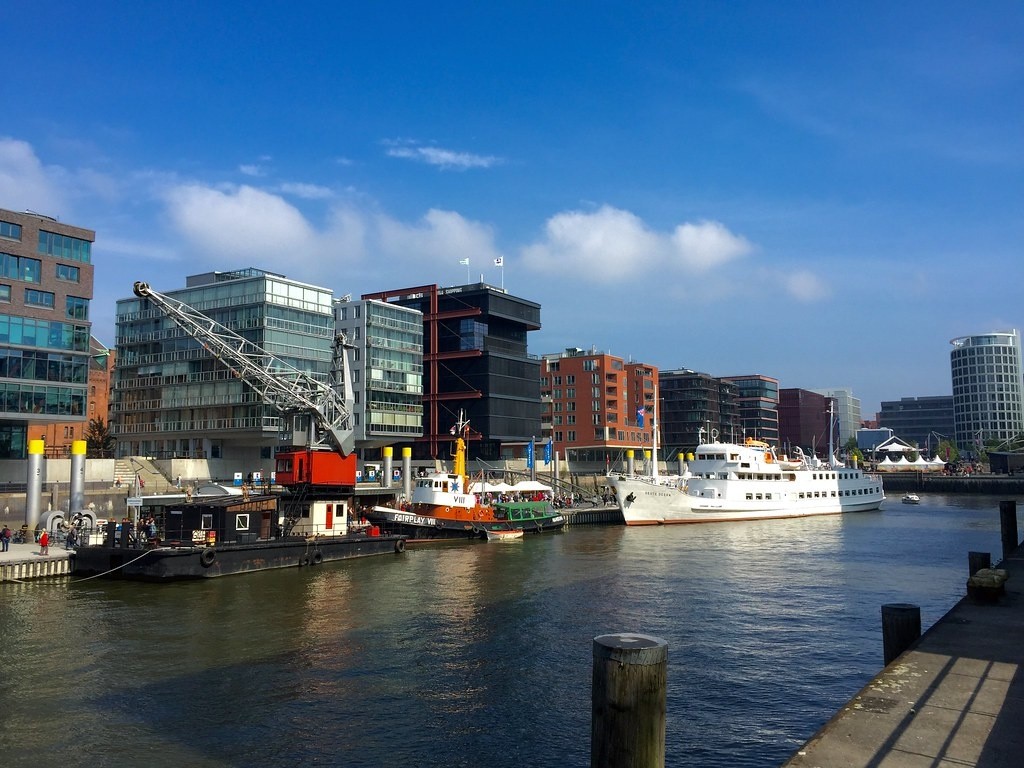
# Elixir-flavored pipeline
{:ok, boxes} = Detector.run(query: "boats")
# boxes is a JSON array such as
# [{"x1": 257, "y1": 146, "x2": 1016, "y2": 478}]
[
  {"x1": 369, "y1": 408, "x2": 566, "y2": 541},
  {"x1": 65, "y1": 282, "x2": 409, "y2": 580},
  {"x1": 486, "y1": 529, "x2": 523, "y2": 540},
  {"x1": 603, "y1": 399, "x2": 886, "y2": 526},
  {"x1": 902, "y1": 491, "x2": 920, "y2": 504}
]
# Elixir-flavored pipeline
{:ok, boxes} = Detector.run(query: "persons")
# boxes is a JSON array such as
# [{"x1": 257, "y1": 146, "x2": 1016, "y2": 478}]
[
  {"x1": 474, "y1": 492, "x2": 616, "y2": 509},
  {"x1": 241, "y1": 483, "x2": 247, "y2": 499},
  {"x1": 402, "y1": 503, "x2": 415, "y2": 513},
  {"x1": 177, "y1": 474, "x2": 183, "y2": 489},
  {"x1": 40, "y1": 528, "x2": 49, "y2": 555},
  {"x1": 0, "y1": 524, "x2": 11, "y2": 552},
  {"x1": 247, "y1": 472, "x2": 254, "y2": 486},
  {"x1": 67, "y1": 525, "x2": 79, "y2": 551},
  {"x1": 186, "y1": 485, "x2": 192, "y2": 502},
  {"x1": 943, "y1": 465, "x2": 983, "y2": 477},
  {"x1": 348, "y1": 506, "x2": 367, "y2": 525},
  {"x1": 137, "y1": 518, "x2": 157, "y2": 539},
  {"x1": 905, "y1": 491, "x2": 908, "y2": 498}
]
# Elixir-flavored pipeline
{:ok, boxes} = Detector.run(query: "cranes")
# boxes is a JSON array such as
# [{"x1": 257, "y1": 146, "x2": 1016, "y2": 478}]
[{"x1": 133, "y1": 283, "x2": 357, "y2": 501}]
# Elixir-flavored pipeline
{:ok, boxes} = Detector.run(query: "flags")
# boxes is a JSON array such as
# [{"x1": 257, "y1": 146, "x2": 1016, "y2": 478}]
[
  {"x1": 450, "y1": 425, "x2": 470, "y2": 436},
  {"x1": 544, "y1": 439, "x2": 552, "y2": 466},
  {"x1": 637, "y1": 406, "x2": 644, "y2": 428},
  {"x1": 527, "y1": 440, "x2": 533, "y2": 468}
]
